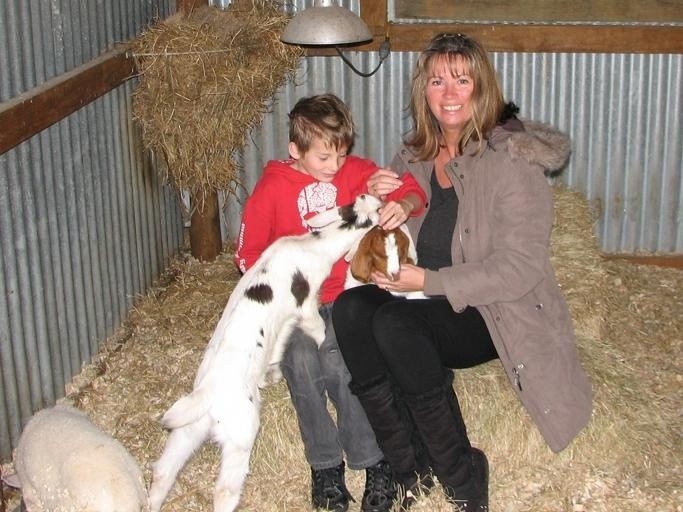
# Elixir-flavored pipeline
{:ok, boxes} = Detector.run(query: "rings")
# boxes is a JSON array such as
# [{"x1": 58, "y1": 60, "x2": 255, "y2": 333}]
[
  {"x1": 385, "y1": 285, "x2": 389, "y2": 290},
  {"x1": 372, "y1": 184, "x2": 376, "y2": 191}
]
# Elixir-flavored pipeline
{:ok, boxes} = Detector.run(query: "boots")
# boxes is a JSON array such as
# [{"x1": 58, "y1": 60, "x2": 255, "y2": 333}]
[
  {"x1": 350, "y1": 375, "x2": 434, "y2": 496},
  {"x1": 401, "y1": 372, "x2": 490, "y2": 503}
]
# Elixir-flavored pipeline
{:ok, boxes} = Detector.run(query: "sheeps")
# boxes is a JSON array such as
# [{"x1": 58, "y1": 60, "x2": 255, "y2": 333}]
[
  {"x1": 0, "y1": 398, "x2": 151, "y2": 512},
  {"x1": 344, "y1": 222, "x2": 430, "y2": 300},
  {"x1": 146, "y1": 194, "x2": 385, "y2": 512}
]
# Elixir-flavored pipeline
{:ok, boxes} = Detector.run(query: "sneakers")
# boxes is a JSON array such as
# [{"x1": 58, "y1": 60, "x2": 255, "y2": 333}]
[
  {"x1": 309, "y1": 460, "x2": 351, "y2": 511},
  {"x1": 360, "y1": 462, "x2": 397, "y2": 512}
]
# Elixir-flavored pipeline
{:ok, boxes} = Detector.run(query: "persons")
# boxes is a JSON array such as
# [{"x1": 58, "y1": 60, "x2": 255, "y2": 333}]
[
  {"x1": 232, "y1": 92, "x2": 429, "y2": 512},
  {"x1": 330, "y1": 32, "x2": 593, "y2": 512}
]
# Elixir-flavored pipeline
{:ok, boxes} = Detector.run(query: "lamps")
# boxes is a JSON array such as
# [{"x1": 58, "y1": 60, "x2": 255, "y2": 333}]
[{"x1": 279, "y1": 0, "x2": 395, "y2": 78}]
